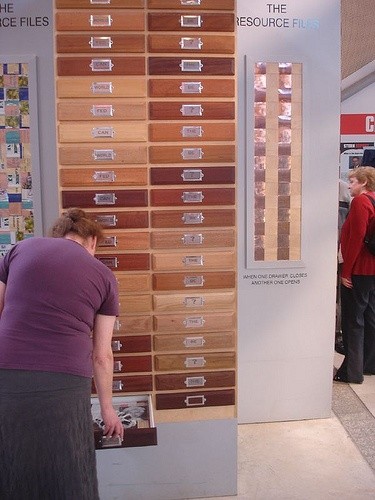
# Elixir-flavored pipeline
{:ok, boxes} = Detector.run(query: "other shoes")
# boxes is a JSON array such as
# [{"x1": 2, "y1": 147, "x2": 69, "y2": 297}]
[
  {"x1": 333, "y1": 375, "x2": 363, "y2": 384},
  {"x1": 335, "y1": 335, "x2": 345, "y2": 355}
]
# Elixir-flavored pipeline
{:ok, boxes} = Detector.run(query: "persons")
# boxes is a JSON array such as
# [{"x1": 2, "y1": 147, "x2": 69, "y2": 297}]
[
  {"x1": 0, "y1": 207, "x2": 124, "y2": 500},
  {"x1": 349, "y1": 156, "x2": 362, "y2": 169},
  {"x1": 332, "y1": 166, "x2": 375, "y2": 383}
]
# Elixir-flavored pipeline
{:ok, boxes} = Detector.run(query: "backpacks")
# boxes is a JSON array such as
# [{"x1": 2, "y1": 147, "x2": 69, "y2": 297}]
[{"x1": 346, "y1": 193, "x2": 375, "y2": 256}]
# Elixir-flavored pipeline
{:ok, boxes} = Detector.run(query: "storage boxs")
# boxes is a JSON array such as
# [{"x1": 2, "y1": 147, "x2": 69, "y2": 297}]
[{"x1": 91, "y1": 393, "x2": 163, "y2": 450}]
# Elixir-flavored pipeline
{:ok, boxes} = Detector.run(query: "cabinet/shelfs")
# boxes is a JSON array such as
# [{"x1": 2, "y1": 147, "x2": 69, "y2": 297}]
[{"x1": 52, "y1": 0, "x2": 239, "y2": 410}]
